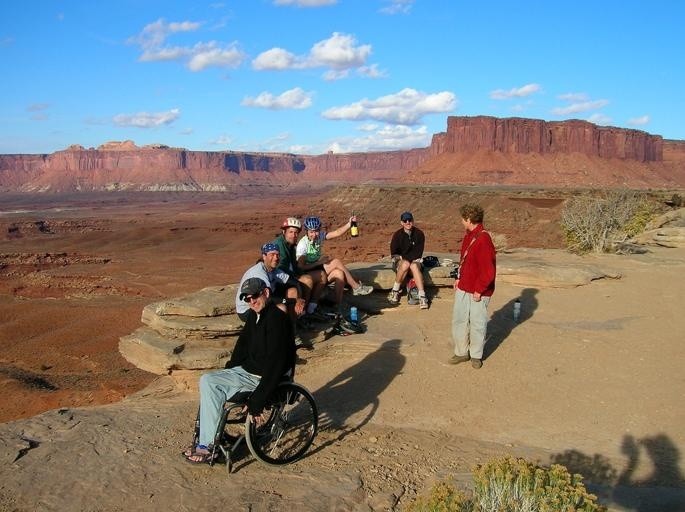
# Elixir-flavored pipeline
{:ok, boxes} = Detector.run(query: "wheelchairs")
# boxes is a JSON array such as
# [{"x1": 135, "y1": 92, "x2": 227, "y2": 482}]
[{"x1": 191, "y1": 338, "x2": 319, "y2": 472}]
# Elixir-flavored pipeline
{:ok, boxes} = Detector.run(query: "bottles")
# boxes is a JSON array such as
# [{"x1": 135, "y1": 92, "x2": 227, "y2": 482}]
[
  {"x1": 350, "y1": 306, "x2": 357, "y2": 321},
  {"x1": 513, "y1": 298, "x2": 522, "y2": 320},
  {"x1": 351, "y1": 209, "x2": 359, "y2": 237}
]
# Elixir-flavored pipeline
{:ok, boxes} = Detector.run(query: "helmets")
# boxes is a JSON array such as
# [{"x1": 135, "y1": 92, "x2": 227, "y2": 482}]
[
  {"x1": 304, "y1": 215, "x2": 322, "y2": 231},
  {"x1": 280, "y1": 217, "x2": 302, "y2": 233},
  {"x1": 334, "y1": 318, "x2": 364, "y2": 335}
]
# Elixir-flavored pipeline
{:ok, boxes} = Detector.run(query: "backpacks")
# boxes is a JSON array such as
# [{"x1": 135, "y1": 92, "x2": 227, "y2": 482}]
[{"x1": 407, "y1": 277, "x2": 428, "y2": 305}]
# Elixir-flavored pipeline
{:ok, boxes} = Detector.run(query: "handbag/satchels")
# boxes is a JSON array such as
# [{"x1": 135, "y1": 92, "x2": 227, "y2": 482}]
[{"x1": 422, "y1": 256, "x2": 438, "y2": 267}]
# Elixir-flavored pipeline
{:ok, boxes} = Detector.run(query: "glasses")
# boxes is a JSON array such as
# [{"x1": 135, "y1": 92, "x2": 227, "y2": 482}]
[{"x1": 244, "y1": 290, "x2": 265, "y2": 302}]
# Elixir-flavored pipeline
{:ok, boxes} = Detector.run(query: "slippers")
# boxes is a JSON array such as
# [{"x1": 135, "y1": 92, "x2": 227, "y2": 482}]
[{"x1": 182, "y1": 442, "x2": 222, "y2": 464}]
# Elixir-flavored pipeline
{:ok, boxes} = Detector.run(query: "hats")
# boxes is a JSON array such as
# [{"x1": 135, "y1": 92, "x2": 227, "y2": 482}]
[
  {"x1": 261, "y1": 243, "x2": 280, "y2": 255},
  {"x1": 239, "y1": 277, "x2": 267, "y2": 301},
  {"x1": 401, "y1": 211, "x2": 413, "y2": 221}
]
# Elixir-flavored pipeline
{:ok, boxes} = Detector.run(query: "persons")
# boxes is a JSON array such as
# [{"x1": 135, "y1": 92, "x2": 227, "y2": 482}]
[
  {"x1": 235, "y1": 217, "x2": 373, "y2": 346},
  {"x1": 181, "y1": 279, "x2": 295, "y2": 465},
  {"x1": 389, "y1": 212, "x2": 430, "y2": 310},
  {"x1": 450, "y1": 204, "x2": 497, "y2": 371}
]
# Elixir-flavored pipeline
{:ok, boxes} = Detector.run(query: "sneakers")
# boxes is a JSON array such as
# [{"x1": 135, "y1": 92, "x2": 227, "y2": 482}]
[
  {"x1": 448, "y1": 352, "x2": 470, "y2": 364},
  {"x1": 352, "y1": 280, "x2": 374, "y2": 296},
  {"x1": 389, "y1": 289, "x2": 399, "y2": 305},
  {"x1": 418, "y1": 295, "x2": 429, "y2": 309},
  {"x1": 471, "y1": 356, "x2": 483, "y2": 369},
  {"x1": 307, "y1": 307, "x2": 334, "y2": 322}
]
